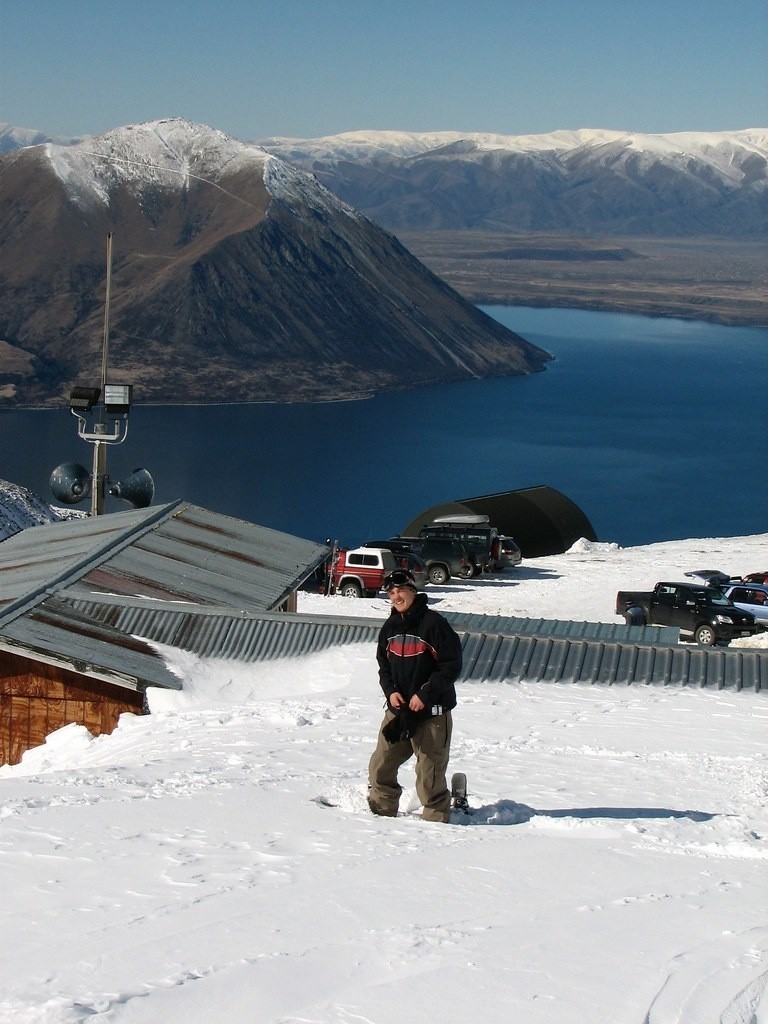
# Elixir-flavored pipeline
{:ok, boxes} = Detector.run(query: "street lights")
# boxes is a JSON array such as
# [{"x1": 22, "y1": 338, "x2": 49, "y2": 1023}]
[{"x1": 70, "y1": 228, "x2": 131, "y2": 514}]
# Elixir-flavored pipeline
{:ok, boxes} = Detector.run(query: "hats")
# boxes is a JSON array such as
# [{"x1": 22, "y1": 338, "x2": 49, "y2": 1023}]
[{"x1": 389, "y1": 570, "x2": 415, "y2": 589}]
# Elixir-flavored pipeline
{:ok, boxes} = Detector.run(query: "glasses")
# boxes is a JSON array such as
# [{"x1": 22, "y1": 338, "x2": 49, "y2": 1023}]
[{"x1": 384, "y1": 572, "x2": 409, "y2": 591}]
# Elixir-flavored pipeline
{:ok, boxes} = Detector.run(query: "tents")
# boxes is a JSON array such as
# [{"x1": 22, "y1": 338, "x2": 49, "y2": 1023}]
[{"x1": 400, "y1": 484, "x2": 598, "y2": 559}]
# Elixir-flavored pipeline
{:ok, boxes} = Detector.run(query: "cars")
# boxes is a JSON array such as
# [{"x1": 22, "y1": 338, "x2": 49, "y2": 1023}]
[{"x1": 741, "y1": 572, "x2": 768, "y2": 585}]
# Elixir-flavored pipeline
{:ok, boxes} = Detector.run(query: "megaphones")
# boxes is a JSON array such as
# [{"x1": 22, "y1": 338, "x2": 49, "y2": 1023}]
[
  {"x1": 49, "y1": 462, "x2": 91, "y2": 505},
  {"x1": 109, "y1": 468, "x2": 155, "y2": 509}
]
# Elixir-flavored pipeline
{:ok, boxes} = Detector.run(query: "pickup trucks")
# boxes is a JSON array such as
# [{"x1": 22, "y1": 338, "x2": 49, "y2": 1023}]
[{"x1": 616, "y1": 581, "x2": 760, "y2": 649}]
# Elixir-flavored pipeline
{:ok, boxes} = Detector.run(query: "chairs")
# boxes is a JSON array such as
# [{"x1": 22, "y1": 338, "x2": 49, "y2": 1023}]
[{"x1": 675, "y1": 589, "x2": 692, "y2": 603}]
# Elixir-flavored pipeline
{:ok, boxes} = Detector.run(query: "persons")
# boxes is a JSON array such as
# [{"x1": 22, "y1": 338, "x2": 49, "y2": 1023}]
[{"x1": 366, "y1": 568, "x2": 463, "y2": 825}]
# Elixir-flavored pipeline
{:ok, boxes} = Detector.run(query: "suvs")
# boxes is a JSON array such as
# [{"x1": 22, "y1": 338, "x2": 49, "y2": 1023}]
[
  {"x1": 682, "y1": 570, "x2": 768, "y2": 620},
  {"x1": 356, "y1": 541, "x2": 428, "y2": 590},
  {"x1": 496, "y1": 534, "x2": 523, "y2": 568},
  {"x1": 311, "y1": 539, "x2": 429, "y2": 599},
  {"x1": 417, "y1": 525, "x2": 503, "y2": 573},
  {"x1": 385, "y1": 535, "x2": 468, "y2": 586}
]
